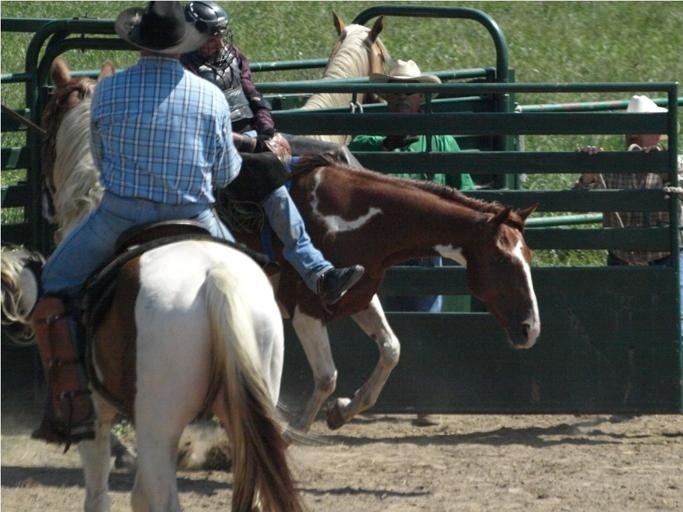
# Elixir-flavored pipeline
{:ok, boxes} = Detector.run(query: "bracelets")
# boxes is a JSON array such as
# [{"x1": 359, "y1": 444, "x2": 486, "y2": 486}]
[{"x1": 238, "y1": 134, "x2": 251, "y2": 155}]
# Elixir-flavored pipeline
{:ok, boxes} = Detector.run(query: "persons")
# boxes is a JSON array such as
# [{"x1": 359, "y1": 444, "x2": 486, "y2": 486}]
[
  {"x1": 572, "y1": 95, "x2": 682, "y2": 269},
  {"x1": 29, "y1": 1, "x2": 243, "y2": 454},
  {"x1": 348, "y1": 58, "x2": 476, "y2": 424},
  {"x1": 176, "y1": 1, "x2": 364, "y2": 306}
]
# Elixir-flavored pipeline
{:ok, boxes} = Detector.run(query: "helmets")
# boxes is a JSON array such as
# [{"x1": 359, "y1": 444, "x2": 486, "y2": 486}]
[{"x1": 184, "y1": 0, "x2": 233, "y2": 68}]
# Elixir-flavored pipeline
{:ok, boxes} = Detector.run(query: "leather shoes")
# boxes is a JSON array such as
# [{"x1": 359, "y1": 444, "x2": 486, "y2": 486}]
[
  {"x1": 29, "y1": 416, "x2": 96, "y2": 440},
  {"x1": 318, "y1": 265, "x2": 364, "y2": 304}
]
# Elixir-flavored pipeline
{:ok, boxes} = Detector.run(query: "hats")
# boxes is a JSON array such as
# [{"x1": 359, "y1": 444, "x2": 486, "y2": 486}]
[
  {"x1": 115, "y1": 1, "x2": 210, "y2": 55},
  {"x1": 614, "y1": 95, "x2": 681, "y2": 141},
  {"x1": 370, "y1": 60, "x2": 442, "y2": 102}
]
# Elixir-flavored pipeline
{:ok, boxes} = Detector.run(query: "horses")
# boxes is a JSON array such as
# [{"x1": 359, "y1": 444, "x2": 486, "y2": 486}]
[
  {"x1": 292, "y1": 8, "x2": 393, "y2": 146},
  {"x1": 40, "y1": 56, "x2": 337, "y2": 512},
  {"x1": 105, "y1": 73, "x2": 540, "y2": 470}
]
